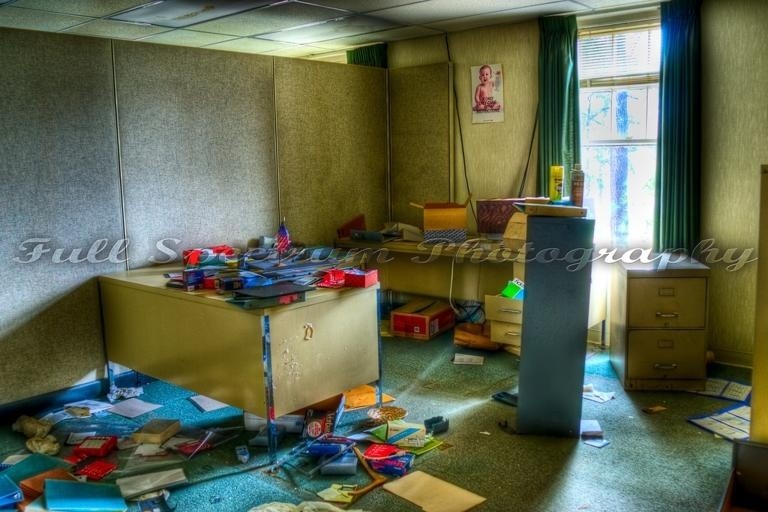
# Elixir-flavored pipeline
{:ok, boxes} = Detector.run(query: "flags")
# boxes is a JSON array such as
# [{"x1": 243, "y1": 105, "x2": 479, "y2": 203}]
[{"x1": 276, "y1": 222, "x2": 290, "y2": 254}]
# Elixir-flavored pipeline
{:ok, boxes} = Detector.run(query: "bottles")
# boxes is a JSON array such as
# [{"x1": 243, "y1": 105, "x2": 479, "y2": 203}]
[{"x1": 570, "y1": 164, "x2": 584, "y2": 207}]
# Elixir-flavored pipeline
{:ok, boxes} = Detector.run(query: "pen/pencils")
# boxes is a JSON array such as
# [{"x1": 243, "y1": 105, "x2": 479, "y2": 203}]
[{"x1": 319, "y1": 432, "x2": 351, "y2": 444}]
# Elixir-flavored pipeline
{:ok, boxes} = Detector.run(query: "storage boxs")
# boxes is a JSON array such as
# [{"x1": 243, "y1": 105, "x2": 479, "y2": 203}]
[{"x1": 417, "y1": 195, "x2": 547, "y2": 244}]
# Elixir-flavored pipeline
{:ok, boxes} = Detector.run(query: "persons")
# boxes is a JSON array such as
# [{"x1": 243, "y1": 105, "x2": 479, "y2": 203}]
[{"x1": 472, "y1": 65, "x2": 504, "y2": 111}]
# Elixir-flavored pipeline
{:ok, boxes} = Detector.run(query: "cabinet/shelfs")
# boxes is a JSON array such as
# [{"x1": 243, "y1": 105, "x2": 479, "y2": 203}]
[
  {"x1": 339, "y1": 209, "x2": 717, "y2": 441},
  {"x1": 99, "y1": 258, "x2": 378, "y2": 469}
]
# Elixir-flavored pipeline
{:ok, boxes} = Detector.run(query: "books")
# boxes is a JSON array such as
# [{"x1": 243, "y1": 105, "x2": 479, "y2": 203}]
[{"x1": 116, "y1": 418, "x2": 188, "y2": 500}]
